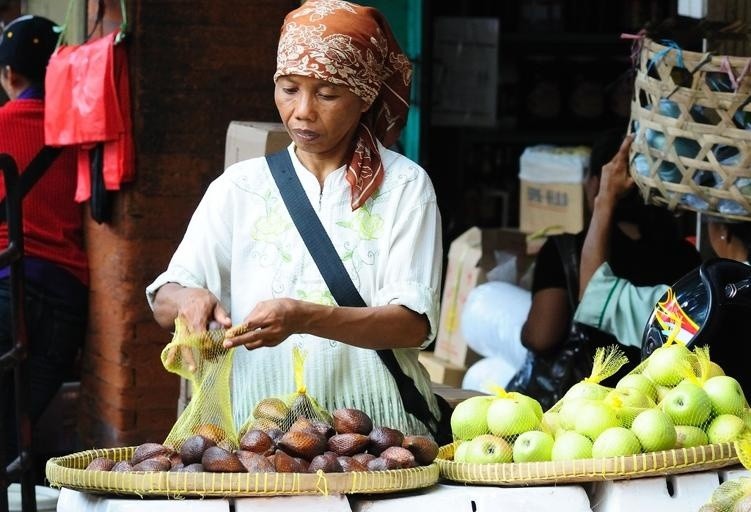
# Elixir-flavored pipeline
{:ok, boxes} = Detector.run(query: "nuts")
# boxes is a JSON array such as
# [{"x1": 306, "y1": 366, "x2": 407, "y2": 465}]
[{"x1": 85, "y1": 394, "x2": 439, "y2": 473}]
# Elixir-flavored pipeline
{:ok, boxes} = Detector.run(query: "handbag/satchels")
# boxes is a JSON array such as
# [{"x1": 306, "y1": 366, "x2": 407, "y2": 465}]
[{"x1": 504, "y1": 327, "x2": 625, "y2": 410}]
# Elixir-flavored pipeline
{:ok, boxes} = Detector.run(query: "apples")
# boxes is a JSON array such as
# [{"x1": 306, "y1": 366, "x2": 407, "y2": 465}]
[{"x1": 450, "y1": 346, "x2": 746, "y2": 464}]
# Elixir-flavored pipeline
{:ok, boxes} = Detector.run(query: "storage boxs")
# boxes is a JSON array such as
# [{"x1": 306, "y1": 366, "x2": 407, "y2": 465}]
[
  {"x1": 432, "y1": 225, "x2": 528, "y2": 369},
  {"x1": 417, "y1": 351, "x2": 466, "y2": 389},
  {"x1": 224, "y1": 121, "x2": 293, "y2": 169},
  {"x1": 519, "y1": 145, "x2": 592, "y2": 236}
]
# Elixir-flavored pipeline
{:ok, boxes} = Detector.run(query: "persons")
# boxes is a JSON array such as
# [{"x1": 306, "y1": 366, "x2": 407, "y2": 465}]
[
  {"x1": 571, "y1": 133, "x2": 750, "y2": 409},
  {"x1": 2, "y1": 14, "x2": 91, "y2": 459},
  {"x1": 143, "y1": 0, "x2": 448, "y2": 446},
  {"x1": 512, "y1": 125, "x2": 702, "y2": 413}
]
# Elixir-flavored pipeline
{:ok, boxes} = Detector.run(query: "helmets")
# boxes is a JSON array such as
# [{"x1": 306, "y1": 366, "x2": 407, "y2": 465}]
[{"x1": 639, "y1": 259, "x2": 751, "y2": 403}]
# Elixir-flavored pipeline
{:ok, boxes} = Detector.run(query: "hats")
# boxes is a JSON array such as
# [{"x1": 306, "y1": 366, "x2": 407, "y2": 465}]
[{"x1": 0, "y1": 14, "x2": 67, "y2": 71}]
